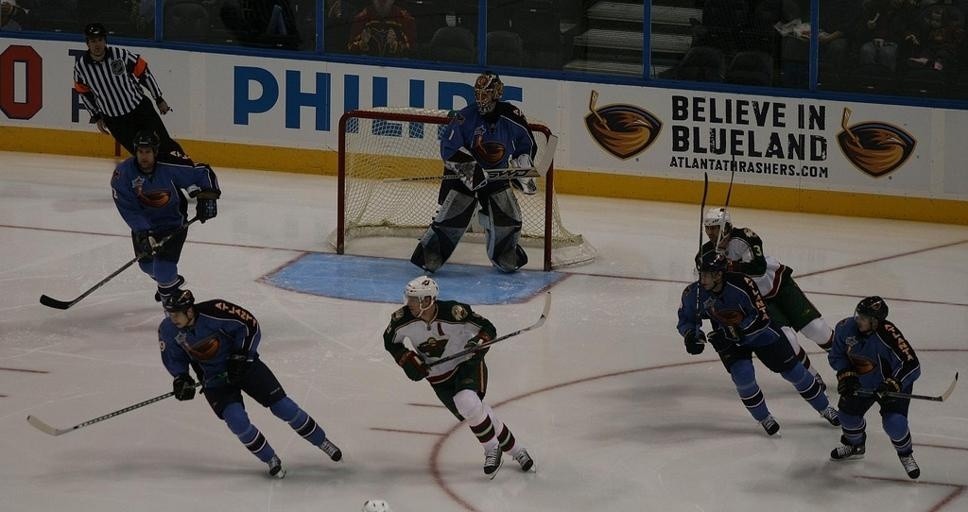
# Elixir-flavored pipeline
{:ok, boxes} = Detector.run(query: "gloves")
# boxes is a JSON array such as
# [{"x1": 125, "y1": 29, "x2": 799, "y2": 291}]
[
  {"x1": 837, "y1": 367, "x2": 861, "y2": 400},
  {"x1": 873, "y1": 376, "x2": 902, "y2": 406},
  {"x1": 684, "y1": 329, "x2": 706, "y2": 355},
  {"x1": 137, "y1": 230, "x2": 158, "y2": 257},
  {"x1": 464, "y1": 333, "x2": 491, "y2": 362},
  {"x1": 174, "y1": 374, "x2": 195, "y2": 400},
  {"x1": 197, "y1": 189, "x2": 221, "y2": 223},
  {"x1": 227, "y1": 350, "x2": 253, "y2": 385},
  {"x1": 397, "y1": 350, "x2": 430, "y2": 382},
  {"x1": 707, "y1": 326, "x2": 738, "y2": 352}
]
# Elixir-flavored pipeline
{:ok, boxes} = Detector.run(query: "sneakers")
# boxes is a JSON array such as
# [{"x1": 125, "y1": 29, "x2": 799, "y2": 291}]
[
  {"x1": 831, "y1": 443, "x2": 866, "y2": 459},
  {"x1": 512, "y1": 447, "x2": 533, "y2": 472},
  {"x1": 758, "y1": 412, "x2": 780, "y2": 436},
  {"x1": 317, "y1": 436, "x2": 342, "y2": 462},
  {"x1": 267, "y1": 452, "x2": 282, "y2": 477},
  {"x1": 897, "y1": 451, "x2": 919, "y2": 478},
  {"x1": 814, "y1": 372, "x2": 826, "y2": 392},
  {"x1": 483, "y1": 444, "x2": 503, "y2": 474},
  {"x1": 186, "y1": 184, "x2": 200, "y2": 198},
  {"x1": 818, "y1": 404, "x2": 841, "y2": 426}
]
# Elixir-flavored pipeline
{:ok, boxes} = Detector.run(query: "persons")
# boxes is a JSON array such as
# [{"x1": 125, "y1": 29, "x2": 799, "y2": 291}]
[
  {"x1": 382, "y1": 273, "x2": 533, "y2": 476},
  {"x1": 409, "y1": 71, "x2": 538, "y2": 274},
  {"x1": 827, "y1": 295, "x2": 920, "y2": 480},
  {"x1": 158, "y1": 291, "x2": 342, "y2": 476},
  {"x1": 695, "y1": 207, "x2": 835, "y2": 405},
  {"x1": 73, "y1": 22, "x2": 200, "y2": 199},
  {"x1": 0, "y1": 0, "x2": 968, "y2": 102},
  {"x1": 677, "y1": 251, "x2": 843, "y2": 436},
  {"x1": 359, "y1": 499, "x2": 391, "y2": 512},
  {"x1": 110, "y1": 127, "x2": 221, "y2": 303}
]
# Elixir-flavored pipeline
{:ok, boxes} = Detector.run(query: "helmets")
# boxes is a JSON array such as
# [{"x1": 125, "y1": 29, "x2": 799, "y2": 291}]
[
  {"x1": 403, "y1": 275, "x2": 440, "y2": 307},
  {"x1": 703, "y1": 207, "x2": 731, "y2": 227},
  {"x1": 694, "y1": 250, "x2": 729, "y2": 285},
  {"x1": 85, "y1": 26, "x2": 105, "y2": 40},
  {"x1": 164, "y1": 290, "x2": 195, "y2": 313},
  {"x1": 133, "y1": 129, "x2": 160, "y2": 154},
  {"x1": 475, "y1": 73, "x2": 504, "y2": 108},
  {"x1": 853, "y1": 296, "x2": 889, "y2": 327}
]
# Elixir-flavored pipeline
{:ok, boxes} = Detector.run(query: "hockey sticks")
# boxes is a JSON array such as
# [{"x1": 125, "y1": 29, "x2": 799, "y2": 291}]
[
  {"x1": 26, "y1": 371, "x2": 233, "y2": 437},
  {"x1": 698, "y1": 140, "x2": 735, "y2": 341},
  {"x1": 40, "y1": 211, "x2": 199, "y2": 309},
  {"x1": 384, "y1": 133, "x2": 558, "y2": 183},
  {"x1": 424, "y1": 292, "x2": 551, "y2": 368},
  {"x1": 854, "y1": 372, "x2": 958, "y2": 402}
]
